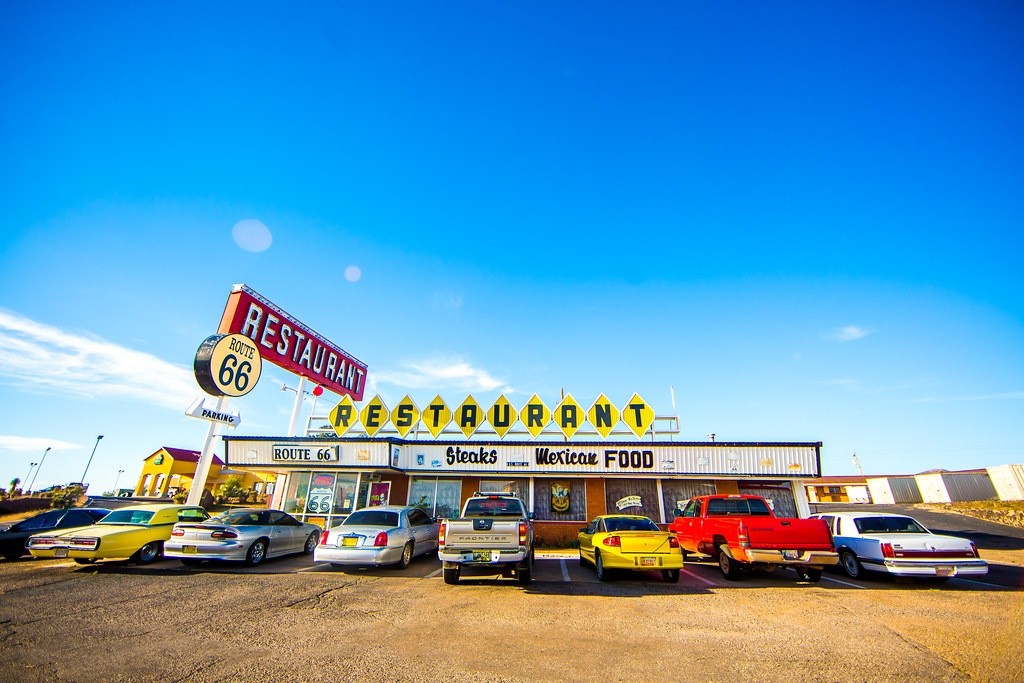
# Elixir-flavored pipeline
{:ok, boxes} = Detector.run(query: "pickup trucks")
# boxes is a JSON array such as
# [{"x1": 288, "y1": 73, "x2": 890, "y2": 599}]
[
  {"x1": 438, "y1": 489, "x2": 535, "y2": 584},
  {"x1": 668, "y1": 495, "x2": 840, "y2": 582}
]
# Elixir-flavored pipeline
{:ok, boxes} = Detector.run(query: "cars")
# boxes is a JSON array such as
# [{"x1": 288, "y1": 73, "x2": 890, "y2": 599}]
[
  {"x1": 807, "y1": 510, "x2": 989, "y2": 580},
  {"x1": 162, "y1": 508, "x2": 323, "y2": 568},
  {"x1": 0, "y1": 509, "x2": 114, "y2": 561},
  {"x1": 577, "y1": 515, "x2": 683, "y2": 583},
  {"x1": 25, "y1": 503, "x2": 211, "y2": 566},
  {"x1": 312, "y1": 506, "x2": 439, "y2": 570}
]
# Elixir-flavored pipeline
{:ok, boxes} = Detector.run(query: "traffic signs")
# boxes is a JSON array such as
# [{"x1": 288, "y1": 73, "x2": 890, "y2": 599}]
[{"x1": 184, "y1": 397, "x2": 241, "y2": 431}]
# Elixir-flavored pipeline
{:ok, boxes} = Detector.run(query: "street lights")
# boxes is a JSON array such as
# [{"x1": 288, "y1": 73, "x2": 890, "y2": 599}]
[
  {"x1": 20, "y1": 462, "x2": 37, "y2": 495},
  {"x1": 26, "y1": 447, "x2": 51, "y2": 496},
  {"x1": 81, "y1": 435, "x2": 103, "y2": 484},
  {"x1": 113, "y1": 469, "x2": 125, "y2": 496}
]
